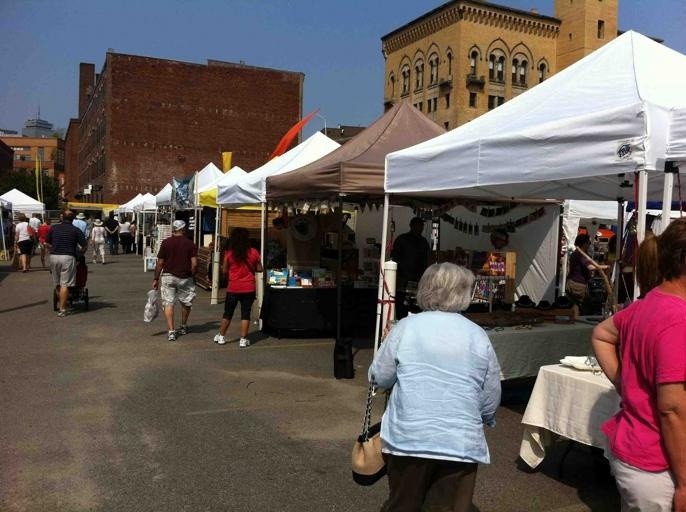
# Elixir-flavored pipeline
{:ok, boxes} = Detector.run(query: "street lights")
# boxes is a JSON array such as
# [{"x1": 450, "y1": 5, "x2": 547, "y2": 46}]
[{"x1": 317, "y1": 113, "x2": 328, "y2": 135}]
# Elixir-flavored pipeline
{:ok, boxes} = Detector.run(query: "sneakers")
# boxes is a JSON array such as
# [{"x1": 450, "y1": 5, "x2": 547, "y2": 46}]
[
  {"x1": 178, "y1": 326, "x2": 187, "y2": 335},
  {"x1": 168, "y1": 329, "x2": 176, "y2": 341},
  {"x1": 213, "y1": 334, "x2": 225, "y2": 345},
  {"x1": 239, "y1": 338, "x2": 250, "y2": 347}
]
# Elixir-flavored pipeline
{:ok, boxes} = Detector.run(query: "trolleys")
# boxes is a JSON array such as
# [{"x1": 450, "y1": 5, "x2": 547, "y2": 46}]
[{"x1": 54, "y1": 247, "x2": 88, "y2": 310}]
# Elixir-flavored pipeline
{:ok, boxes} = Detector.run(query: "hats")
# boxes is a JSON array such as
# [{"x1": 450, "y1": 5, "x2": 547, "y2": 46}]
[
  {"x1": 93, "y1": 219, "x2": 103, "y2": 225},
  {"x1": 75, "y1": 213, "x2": 85, "y2": 219},
  {"x1": 171, "y1": 219, "x2": 186, "y2": 232}
]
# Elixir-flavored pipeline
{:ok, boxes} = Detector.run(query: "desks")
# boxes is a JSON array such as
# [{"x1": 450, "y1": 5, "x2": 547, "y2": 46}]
[
  {"x1": 259, "y1": 276, "x2": 512, "y2": 340},
  {"x1": 483, "y1": 311, "x2": 607, "y2": 382},
  {"x1": 518, "y1": 355, "x2": 623, "y2": 469}
]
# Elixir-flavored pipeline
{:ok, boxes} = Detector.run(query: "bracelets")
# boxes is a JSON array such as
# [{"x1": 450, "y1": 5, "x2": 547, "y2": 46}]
[{"x1": 154, "y1": 278, "x2": 160, "y2": 280}]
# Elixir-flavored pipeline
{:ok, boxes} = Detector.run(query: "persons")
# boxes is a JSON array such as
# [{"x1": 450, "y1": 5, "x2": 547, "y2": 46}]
[
  {"x1": 393, "y1": 217, "x2": 433, "y2": 319},
  {"x1": 268, "y1": 218, "x2": 287, "y2": 260},
  {"x1": 213, "y1": 228, "x2": 263, "y2": 348},
  {"x1": 14, "y1": 213, "x2": 35, "y2": 272},
  {"x1": 564, "y1": 219, "x2": 640, "y2": 324},
  {"x1": 29, "y1": 210, "x2": 139, "y2": 269},
  {"x1": 45, "y1": 210, "x2": 89, "y2": 317},
  {"x1": 368, "y1": 263, "x2": 502, "y2": 512},
  {"x1": 0, "y1": 210, "x2": 13, "y2": 250},
  {"x1": 590, "y1": 216, "x2": 686, "y2": 512},
  {"x1": 152, "y1": 219, "x2": 199, "y2": 340}
]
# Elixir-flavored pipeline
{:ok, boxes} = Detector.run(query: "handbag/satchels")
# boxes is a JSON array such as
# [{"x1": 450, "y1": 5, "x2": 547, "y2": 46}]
[
  {"x1": 143, "y1": 289, "x2": 158, "y2": 323},
  {"x1": 350, "y1": 374, "x2": 388, "y2": 487},
  {"x1": 26, "y1": 226, "x2": 35, "y2": 237},
  {"x1": 565, "y1": 278, "x2": 586, "y2": 299}
]
